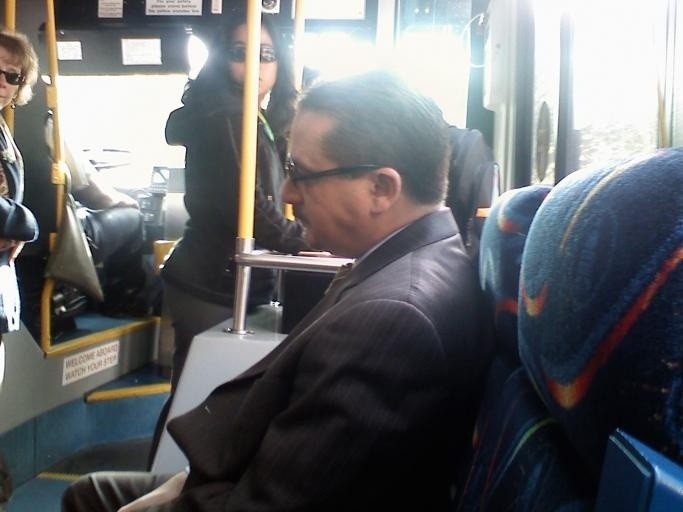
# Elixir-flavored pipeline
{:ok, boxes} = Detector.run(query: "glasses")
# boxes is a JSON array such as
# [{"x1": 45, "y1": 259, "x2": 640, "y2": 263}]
[
  {"x1": 226, "y1": 44, "x2": 278, "y2": 63},
  {"x1": 0, "y1": 69, "x2": 25, "y2": 86},
  {"x1": 286, "y1": 165, "x2": 388, "y2": 186}
]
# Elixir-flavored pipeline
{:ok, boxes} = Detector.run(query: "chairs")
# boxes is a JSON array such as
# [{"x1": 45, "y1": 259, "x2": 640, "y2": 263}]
[
  {"x1": 481, "y1": 145, "x2": 683, "y2": 511},
  {"x1": 452, "y1": 186, "x2": 552, "y2": 511},
  {"x1": 12, "y1": 77, "x2": 87, "y2": 340}
]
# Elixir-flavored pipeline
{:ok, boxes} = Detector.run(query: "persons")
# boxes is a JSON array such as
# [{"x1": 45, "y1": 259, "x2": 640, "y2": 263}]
[
  {"x1": 61, "y1": 71, "x2": 483, "y2": 512},
  {"x1": 0, "y1": 32, "x2": 44, "y2": 332},
  {"x1": 44, "y1": 115, "x2": 161, "y2": 315},
  {"x1": 146, "y1": 11, "x2": 317, "y2": 474}
]
